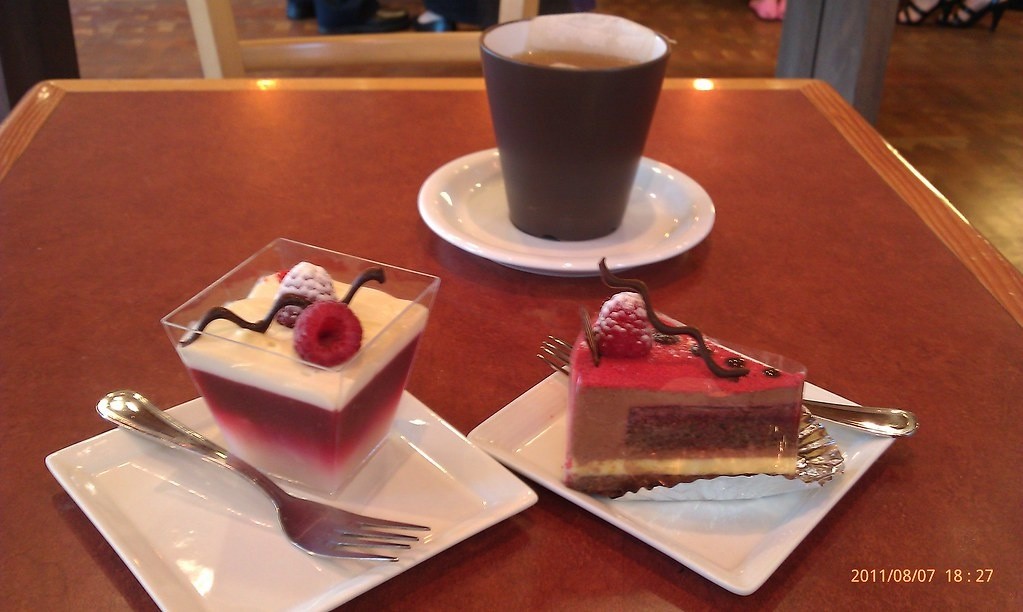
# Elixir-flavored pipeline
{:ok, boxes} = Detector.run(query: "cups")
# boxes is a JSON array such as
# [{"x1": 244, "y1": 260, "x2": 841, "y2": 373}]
[
  {"x1": 161, "y1": 238, "x2": 440, "y2": 498},
  {"x1": 479, "y1": 19, "x2": 671, "y2": 242}
]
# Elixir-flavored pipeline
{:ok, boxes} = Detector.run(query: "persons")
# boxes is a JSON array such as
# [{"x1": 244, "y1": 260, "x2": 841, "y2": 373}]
[
  {"x1": 286, "y1": 0, "x2": 592, "y2": 30},
  {"x1": 897, "y1": 0, "x2": 1008, "y2": 30}
]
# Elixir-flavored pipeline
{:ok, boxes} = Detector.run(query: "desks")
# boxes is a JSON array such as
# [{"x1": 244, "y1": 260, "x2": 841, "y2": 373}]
[{"x1": 0, "y1": 79, "x2": 1022, "y2": 612}]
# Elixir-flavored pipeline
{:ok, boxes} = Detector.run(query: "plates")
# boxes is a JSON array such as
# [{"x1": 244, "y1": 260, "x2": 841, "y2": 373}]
[
  {"x1": 418, "y1": 149, "x2": 715, "y2": 275},
  {"x1": 45, "y1": 390, "x2": 537, "y2": 612},
  {"x1": 467, "y1": 343, "x2": 896, "y2": 595}
]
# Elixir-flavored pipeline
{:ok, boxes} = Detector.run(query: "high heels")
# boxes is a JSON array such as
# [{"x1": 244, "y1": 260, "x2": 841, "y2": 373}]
[
  {"x1": 937, "y1": 0, "x2": 1008, "y2": 32},
  {"x1": 898, "y1": 0, "x2": 954, "y2": 26}
]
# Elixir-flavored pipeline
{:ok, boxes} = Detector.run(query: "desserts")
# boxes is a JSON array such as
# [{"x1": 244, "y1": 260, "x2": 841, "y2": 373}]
[
  {"x1": 564, "y1": 257, "x2": 804, "y2": 499},
  {"x1": 174, "y1": 259, "x2": 428, "y2": 497}
]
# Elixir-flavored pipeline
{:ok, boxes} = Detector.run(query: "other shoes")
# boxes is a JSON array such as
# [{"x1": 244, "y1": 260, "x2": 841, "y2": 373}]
[
  {"x1": 331, "y1": 11, "x2": 409, "y2": 34},
  {"x1": 412, "y1": 13, "x2": 456, "y2": 33},
  {"x1": 286, "y1": 0, "x2": 314, "y2": 19}
]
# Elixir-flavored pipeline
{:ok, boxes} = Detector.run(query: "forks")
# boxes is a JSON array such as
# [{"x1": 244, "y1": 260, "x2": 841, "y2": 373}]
[
  {"x1": 97, "y1": 390, "x2": 434, "y2": 562},
  {"x1": 538, "y1": 335, "x2": 919, "y2": 436}
]
